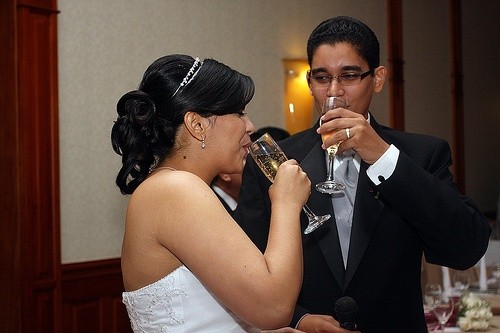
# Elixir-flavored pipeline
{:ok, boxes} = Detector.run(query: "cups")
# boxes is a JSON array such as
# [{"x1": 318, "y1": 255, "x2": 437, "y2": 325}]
[
  {"x1": 425, "y1": 284, "x2": 442, "y2": 310},
  {"x1": 453, "y1": 271, "x2": 471, "y2": 290}
]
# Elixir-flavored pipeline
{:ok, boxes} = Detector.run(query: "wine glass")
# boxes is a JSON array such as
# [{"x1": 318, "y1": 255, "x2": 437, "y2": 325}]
[
  {"x1": 246, "y1": 132, "x2": 331, "y2": 234},
  {"x1": 431, "y1": 295, "x2": 454, "y2": 333},
  {"x1": 315, "y1": 97, "x2": 346, "y2": 193}
]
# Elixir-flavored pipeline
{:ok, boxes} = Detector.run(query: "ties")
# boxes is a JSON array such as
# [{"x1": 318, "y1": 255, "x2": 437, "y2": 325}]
[{"x1": 331, "y1": 148, "x2": 360, "y2": 270}]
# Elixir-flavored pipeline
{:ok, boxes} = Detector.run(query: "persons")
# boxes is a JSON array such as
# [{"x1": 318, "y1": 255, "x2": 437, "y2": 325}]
[
  {"x1": 111, "y1": 53, "x2": 311, "y2": 333},
  {"x1": 234, "y1": 16, "x2": 492, "y2": 333},
  {"x1": 209, "y1": 124, "x2": 291, "y2": 218}
]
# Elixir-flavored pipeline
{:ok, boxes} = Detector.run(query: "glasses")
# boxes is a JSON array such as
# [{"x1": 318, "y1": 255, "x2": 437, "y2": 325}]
[{"x1": 309, "y1": 69, "x2": 375, "y2": 85}]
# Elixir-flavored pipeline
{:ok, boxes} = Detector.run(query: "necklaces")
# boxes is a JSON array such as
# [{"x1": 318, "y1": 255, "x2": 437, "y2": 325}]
[{"x1": 153, "y1": 166, "x2": 177, "y2": 171}]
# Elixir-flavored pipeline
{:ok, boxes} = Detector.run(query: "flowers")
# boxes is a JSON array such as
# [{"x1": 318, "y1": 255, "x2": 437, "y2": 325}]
[{"x1": 424, "y1": 292, "x2": 493, "y2": 332}]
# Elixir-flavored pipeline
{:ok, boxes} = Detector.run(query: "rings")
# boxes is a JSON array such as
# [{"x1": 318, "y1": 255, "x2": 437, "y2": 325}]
[{"x1": 346, "y1": 128, "x2": 351, "y2": 139}]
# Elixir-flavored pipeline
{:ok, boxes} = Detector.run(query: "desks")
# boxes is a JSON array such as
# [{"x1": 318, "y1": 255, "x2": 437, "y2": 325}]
[{"x1": 423, "y1": 287, "x2": 500, "y2": 333}]
